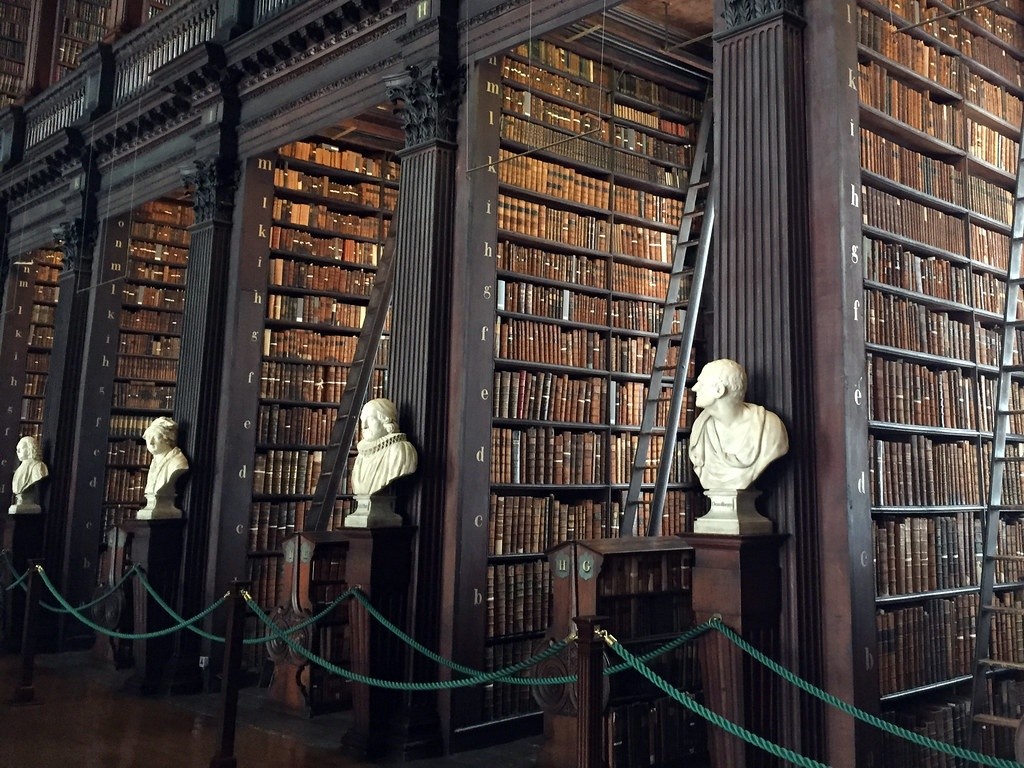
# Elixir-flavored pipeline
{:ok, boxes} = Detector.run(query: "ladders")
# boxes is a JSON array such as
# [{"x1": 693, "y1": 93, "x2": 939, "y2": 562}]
[
  {"x1": 967, "y1": 102, "x2": 1024, "y2": 768},
  {"x1": 304, "y1": 187, "x2": 400, "y2": 533},
  {"x1": 617, "y1": 80, "x2": 717, "y2": 539}
]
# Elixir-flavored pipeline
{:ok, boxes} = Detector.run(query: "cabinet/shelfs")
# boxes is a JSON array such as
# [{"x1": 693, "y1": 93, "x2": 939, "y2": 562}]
[{"x1": 0, "y1": 0, "x2": 1024, "y2": 768}]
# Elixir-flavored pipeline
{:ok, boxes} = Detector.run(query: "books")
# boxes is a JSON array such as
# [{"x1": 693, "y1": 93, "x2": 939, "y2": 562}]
[
  {"x1": 55, "y1": 0, "x2": 112, "y2": 81},
  {"x1": 148, "y1": 0, "x2": 175, "y2": 19},
  {"x1": 98, "y1": 201, "x2": 195, "y2": 587},
  {"x1": 18, "y1": 250, "x2": 64, "y2": 443},
  {"x1": 856, "y1": 1, "x2": 1024, "y2": 768},
  {"x1": 0, "y1": 0, "x2": 31, "y2": 109},
  {"x1": 245, "y1": 142, "x2": 401, "y2": 666},
  {"x1": 483, "y1": 38, "x2": 703, "y2": 722}
]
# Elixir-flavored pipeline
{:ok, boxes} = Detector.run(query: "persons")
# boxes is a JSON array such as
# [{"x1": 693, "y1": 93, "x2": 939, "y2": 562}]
[
  {"x1": 13, "y1": 436, "x2": 49, "y2": 494},
  {"x1": 350, "y1": 398, "x2": 418, "y2": 495},
  {"x1": 142, "y1": 416, "x2": 189, "y2": 495},
  {"x1": 688, "y1": 358, "x2": 789, "y2": 490}
]
